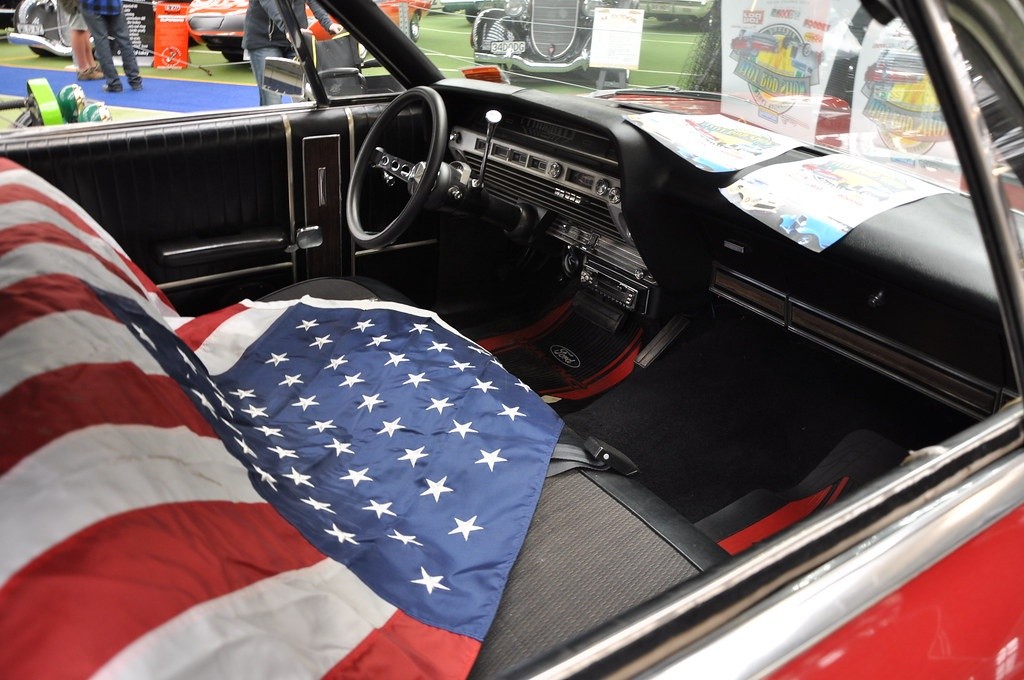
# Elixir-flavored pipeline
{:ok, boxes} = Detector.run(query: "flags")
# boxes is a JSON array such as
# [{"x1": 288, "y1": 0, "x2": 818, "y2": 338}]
[{"x1": 1, "y1": 157, "x2": 563, "y2": 679}]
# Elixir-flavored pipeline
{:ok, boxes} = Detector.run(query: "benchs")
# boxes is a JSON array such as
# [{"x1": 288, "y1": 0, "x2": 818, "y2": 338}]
[{"x1": 0, "y1": 157, "x2": 736, "y2": 680}]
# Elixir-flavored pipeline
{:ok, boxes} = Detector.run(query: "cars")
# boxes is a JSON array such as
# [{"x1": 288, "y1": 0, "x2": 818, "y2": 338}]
[
  {"x1": 0, "y1": 0, "x2": 720, "y2": 87},
  {"x1": 0, "y1": 1, "x2": 1024, "y2": 679}
]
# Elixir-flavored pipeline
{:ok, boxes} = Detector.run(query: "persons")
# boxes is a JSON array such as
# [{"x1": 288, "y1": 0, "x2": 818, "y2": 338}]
[
  {"x1": 241, "y1": 0, "x2": 343, "y2": 105},
  {"x1": 58, "y1": 0, "x2": 143, "y2": 92}
]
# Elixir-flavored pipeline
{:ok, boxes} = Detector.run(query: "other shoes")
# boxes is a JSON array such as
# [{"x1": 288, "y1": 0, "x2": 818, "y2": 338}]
[
  {"x1": 77, "y1": 68, "x2": 104, "y2": 80},
  {"x1": 103, "y1": 85, "x2": 122, "y2": 92},
  {"x1": 92, "y1": 64, "x2": 103, "y2": 72},
  {"x1": 134, "y1": 85, "x2": 142, "y2": 91}
]
